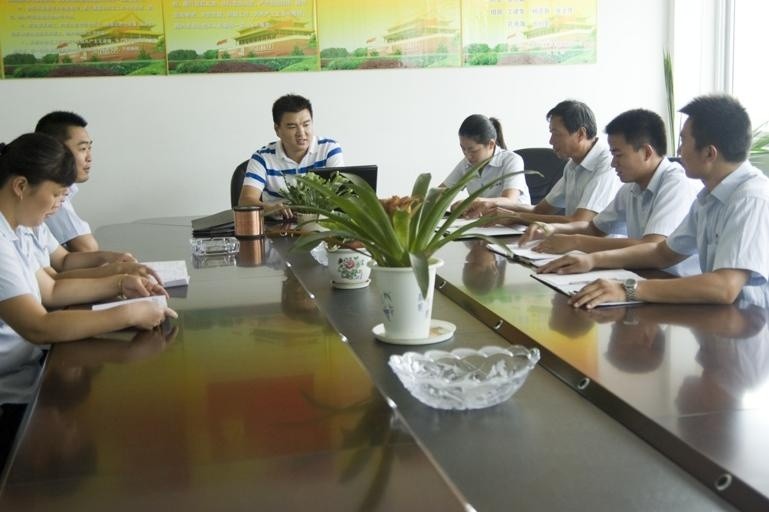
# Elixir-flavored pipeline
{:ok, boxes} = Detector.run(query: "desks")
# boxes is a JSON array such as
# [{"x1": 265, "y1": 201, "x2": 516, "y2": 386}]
[{"x1": 0, "y1": 213, "x2": 769, "y2": 512}]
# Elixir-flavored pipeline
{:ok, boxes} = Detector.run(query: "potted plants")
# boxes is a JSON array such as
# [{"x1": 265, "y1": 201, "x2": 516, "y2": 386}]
[{"x1": 278, "y1": 168, "x2": 353, "y2": 232}]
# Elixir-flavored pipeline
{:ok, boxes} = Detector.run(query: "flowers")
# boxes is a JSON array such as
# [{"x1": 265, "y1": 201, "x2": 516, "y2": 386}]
[{"x1": 283, "y1": 151, "x2": 544, "y2": 300}]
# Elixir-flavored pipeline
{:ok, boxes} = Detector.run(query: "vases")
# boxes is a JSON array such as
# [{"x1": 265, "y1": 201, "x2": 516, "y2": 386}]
[
  {"x1": 327, "y1": 247, "x2": 372, "y2": 285},
  {"x1": 363, "y1": 256, "x2": 444, "y2": 339}
]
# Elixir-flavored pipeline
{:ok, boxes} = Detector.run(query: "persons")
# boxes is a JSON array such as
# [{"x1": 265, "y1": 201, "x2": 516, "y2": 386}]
[
  {"x1": 548, "y1": 289, "x2": 595, "y2": 338},
  {"x1": 536, "y1": 91, "x2": 769, "y2": 308},
  {"x1": 584, "y1": 307, "x2": 768, "y2": 454},
  {"x1": 462, "y1": 236, "x2": 502, "y2": 294},
  {"x1": 603, "y1": 319, "x2": 665, "y2": 374},
  {"x1": 451, "y1": 99, "x2": 624, "y2": 228},
  {"x1": 423, "y1": 93, "x2": 532, "y2": 222},
  {"x1": 518, "y1": 108, "x2": 703, "y2": 278},
  {"x1": 238, "y1": 93, "x2": 349, "y2": 228}
]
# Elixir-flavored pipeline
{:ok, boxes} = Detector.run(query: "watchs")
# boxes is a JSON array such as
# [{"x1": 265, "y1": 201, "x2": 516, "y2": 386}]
[
  {"x1": 624, "y1": 310, "x2": 644, "y2": 326},
  {"x1": 623, "y1": 276, "x2": 641, "y2": 298}
]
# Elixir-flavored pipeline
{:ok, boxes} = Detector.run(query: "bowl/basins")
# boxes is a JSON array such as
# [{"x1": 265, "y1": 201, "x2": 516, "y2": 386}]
[{"x1": 387, "y1": 343, "x2": 543, "y2": 411}]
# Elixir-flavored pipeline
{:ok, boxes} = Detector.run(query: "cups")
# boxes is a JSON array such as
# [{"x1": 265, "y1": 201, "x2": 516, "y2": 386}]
[
  {"x1": 234, "y1": 206, "x2": 263, "y2": 237},
  {"x1": 236, "y1": 238, "x2": 265, "y2": 267}
]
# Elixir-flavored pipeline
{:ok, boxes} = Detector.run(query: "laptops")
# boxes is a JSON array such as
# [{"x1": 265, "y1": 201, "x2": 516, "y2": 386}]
[{"x1": 306, "y1": 165, "x2": 379, "y2": 211}]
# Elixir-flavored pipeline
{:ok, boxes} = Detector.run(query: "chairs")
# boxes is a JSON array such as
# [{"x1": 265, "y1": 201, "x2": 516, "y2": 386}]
[
  {"x1": 512, "y1": 148, "x2": 570, "y2": 206},
  {"x1": 232, "y1": 158, "x2": 262, "y2": 209},
  {"x1": 665, "y1": 153, "x2": 688, "y2": 170}
]
